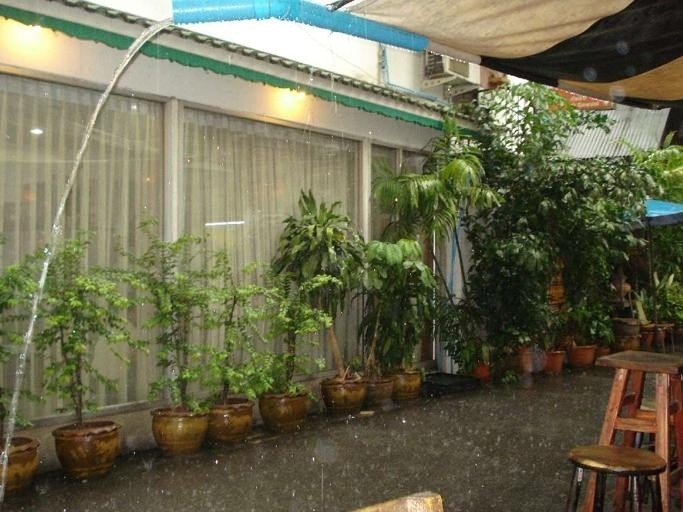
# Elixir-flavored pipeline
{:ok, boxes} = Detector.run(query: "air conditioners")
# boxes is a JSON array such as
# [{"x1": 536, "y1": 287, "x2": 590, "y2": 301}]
[{"x1": 425, "y1": 51, "x2": 480, "y2": 86}]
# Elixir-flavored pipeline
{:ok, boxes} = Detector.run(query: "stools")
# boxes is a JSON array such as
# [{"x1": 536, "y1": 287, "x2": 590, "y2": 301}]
[{"x1": 563, "y1": 444, "x2": 666, "y2": 512}]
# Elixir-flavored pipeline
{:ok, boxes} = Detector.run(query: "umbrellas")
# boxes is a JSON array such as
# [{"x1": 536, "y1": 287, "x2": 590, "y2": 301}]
[{"x1": 621, "y1": 197, "x2": 682, "y2": 325}]
